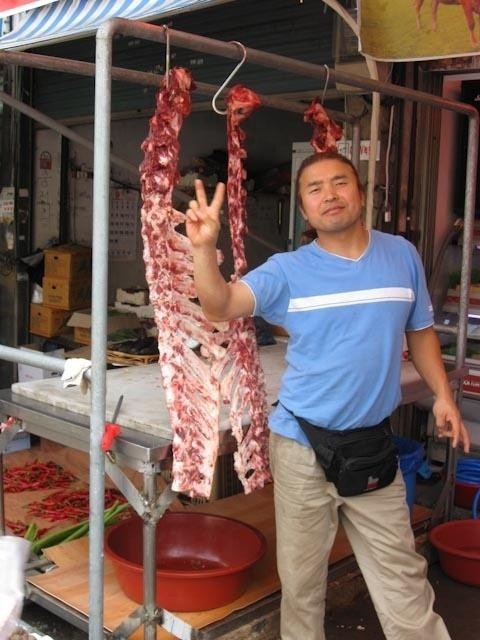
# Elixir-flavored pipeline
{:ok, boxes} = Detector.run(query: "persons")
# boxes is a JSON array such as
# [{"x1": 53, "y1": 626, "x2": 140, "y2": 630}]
[{"x1": 186, "y1": 152, "x2": 470, "y2": 640}]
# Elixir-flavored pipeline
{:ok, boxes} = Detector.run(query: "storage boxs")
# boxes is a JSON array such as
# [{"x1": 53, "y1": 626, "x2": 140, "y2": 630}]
[
  {"x1": 30, "y1": 304, "x2": 70, "y2": 338},
  {"x1": 45, "y1": 246, "x2": 95, "y2": 279},
  {"x1": 43, "y1": 277, "x2": 91, "y2": 310},
  {"x1": 67, "y1": 313, "x2": 142, "y2": 346}
]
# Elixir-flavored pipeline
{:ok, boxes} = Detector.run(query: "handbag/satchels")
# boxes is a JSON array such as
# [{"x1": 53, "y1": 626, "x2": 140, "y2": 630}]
[{"x1": 271, "y1": 398, "x2": 398, "y2": 497}]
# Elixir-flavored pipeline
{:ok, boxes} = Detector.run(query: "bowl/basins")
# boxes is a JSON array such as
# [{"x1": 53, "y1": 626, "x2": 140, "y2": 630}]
[
  {"x1": 429, "y1": 518, "x2": 480, "y2": 585},
  {"x1": 104, "y1": 511, "x2": 268, "y2": 613},
  {"x1": 439, "y1": 469, "x2": 480, "y2": 510}
]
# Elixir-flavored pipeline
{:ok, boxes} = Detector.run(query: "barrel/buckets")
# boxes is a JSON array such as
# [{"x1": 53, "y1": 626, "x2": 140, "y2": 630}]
[{"x1": 397, "y1": 435, "x2": 433, "y2": 515}]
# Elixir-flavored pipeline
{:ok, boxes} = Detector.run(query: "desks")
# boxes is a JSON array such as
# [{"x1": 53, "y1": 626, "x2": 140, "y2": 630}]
[{"x1": 0, "y1": 364, "x2": 472, "y2": 640}]
